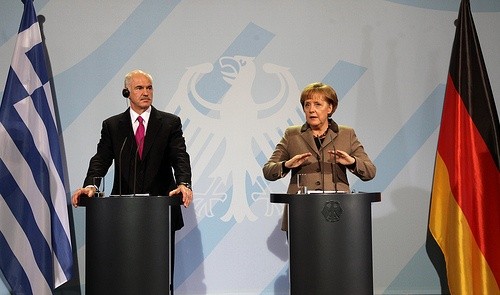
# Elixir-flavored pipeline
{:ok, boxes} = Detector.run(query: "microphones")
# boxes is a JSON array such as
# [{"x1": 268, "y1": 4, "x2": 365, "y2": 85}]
[
  {"x1": 317, "y1": 134, "x2": 325, "y2": 194},
  {"x1": 329, "y1": 134, "x2": 337, "y2": 194},
  {"x1": 119, "y1": 137, "x2": 130, "y2": 197},
  {"x1": 133, "y1": 136, "x2": 143, "y2": 197}
]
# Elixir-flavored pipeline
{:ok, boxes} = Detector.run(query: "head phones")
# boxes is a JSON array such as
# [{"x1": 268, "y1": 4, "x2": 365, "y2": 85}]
[{"x1": 122, "y1": 78, "x2": 130, "y2": 98}]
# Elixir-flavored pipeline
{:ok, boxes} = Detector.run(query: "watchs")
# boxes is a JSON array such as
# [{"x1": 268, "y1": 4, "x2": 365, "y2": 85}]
[{"x1": 178, "y1": 181, "x2": 192, "y2": 191}]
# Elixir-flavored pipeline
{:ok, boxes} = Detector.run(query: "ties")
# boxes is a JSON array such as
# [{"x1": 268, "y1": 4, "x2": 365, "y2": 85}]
[{"x1": 135, "y1": 116, "x2": 145, "y2": 160}]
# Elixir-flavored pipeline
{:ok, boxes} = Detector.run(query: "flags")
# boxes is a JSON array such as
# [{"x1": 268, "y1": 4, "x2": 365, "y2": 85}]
[
  {"x1": 426, "y1": 0, "x2": 500, "y2": 295},
  {"x1": 0, "y1": 0, "x2": 74, "y2": 295}
]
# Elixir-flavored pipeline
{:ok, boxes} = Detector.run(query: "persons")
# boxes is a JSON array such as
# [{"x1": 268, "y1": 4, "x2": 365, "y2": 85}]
[
  {"x1": 263, "y1": 81, "x2": 377, "y2": 242},
  {"x1": 70, "y1": 69, "x2": 194, "y2": 294}
]
